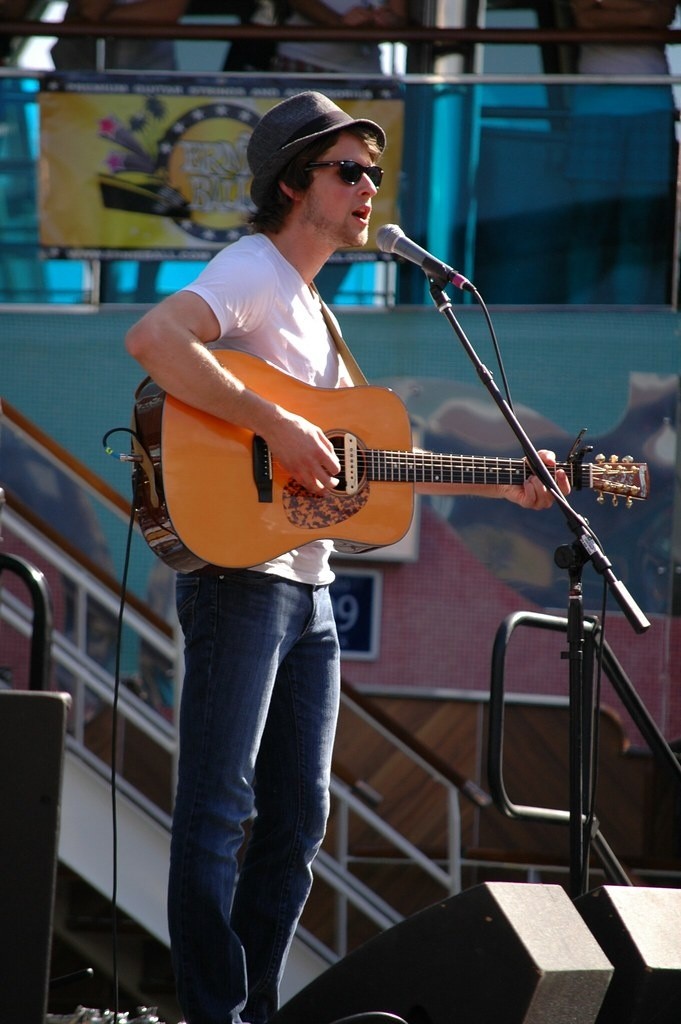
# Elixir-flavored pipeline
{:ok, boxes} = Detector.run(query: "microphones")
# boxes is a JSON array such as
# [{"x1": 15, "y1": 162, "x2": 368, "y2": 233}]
[{"x1": 376, "y1": 223, "x2": 477, "y2": 293}]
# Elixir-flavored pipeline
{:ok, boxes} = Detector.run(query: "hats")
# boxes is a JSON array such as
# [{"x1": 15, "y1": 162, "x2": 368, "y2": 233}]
[{"x1": 246, "y1": 91, "x2": 387, "y2": 207}]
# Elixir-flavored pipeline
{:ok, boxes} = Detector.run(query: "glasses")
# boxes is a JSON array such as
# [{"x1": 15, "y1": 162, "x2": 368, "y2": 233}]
[{"x1": 304, "y1": 159, "x2": 384, "y2": 190}]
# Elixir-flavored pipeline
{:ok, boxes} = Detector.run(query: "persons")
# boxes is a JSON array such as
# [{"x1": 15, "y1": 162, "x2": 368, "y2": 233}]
[
  {"x1": 50, "y1": 0, "x2": 681, "y2": 303},
  {"x1": 126, "y1": 91, "x2": 572, "y2": 1024}
]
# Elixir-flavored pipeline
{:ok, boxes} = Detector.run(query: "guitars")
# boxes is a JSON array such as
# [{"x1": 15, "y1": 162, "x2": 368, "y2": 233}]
[{"x1": 132, "y1": 348, "x2": 650, "y2": 577}]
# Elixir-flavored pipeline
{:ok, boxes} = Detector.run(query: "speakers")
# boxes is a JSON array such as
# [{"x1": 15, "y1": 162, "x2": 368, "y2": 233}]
[
  {"x1": 267, "y1": 880, "x2": 614, "y2": 1024},
  {"x1": 573, "y1": 886, "x2": 681, "y2": 1024},
  {"x1": 0, "y1": 690, "x2": 72, "y2": 1024}
]
